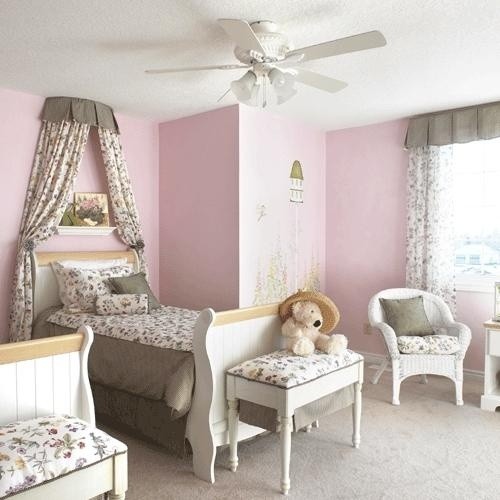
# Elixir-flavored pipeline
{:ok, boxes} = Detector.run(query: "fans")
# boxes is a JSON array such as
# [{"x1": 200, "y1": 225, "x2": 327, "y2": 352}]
[{"x1": 145, "y1": 20, "x2": 386, "y2": 94}]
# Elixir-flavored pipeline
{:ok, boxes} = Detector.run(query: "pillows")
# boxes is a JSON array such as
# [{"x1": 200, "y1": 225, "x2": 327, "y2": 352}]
[
  {"x1": 56, "y1": 263, "x2": 132, "y2": 314},
  {"x1": 108, "y1": 271, "x2": 161, "y2": 310},
  {"x1": 380, "y1": 296, "x2": 435, "y2": 337},
  {"x1": 49, "y1": 256, "x2": 128, "y2": 310},
  {"x1": 95, "y1": 293, "x2": 147, "y2": 314}
]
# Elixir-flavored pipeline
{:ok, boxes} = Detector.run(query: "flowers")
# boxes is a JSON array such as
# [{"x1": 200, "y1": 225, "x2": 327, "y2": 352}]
[{"x1": 77, "y1": 195, "x2": 104, "y2": 223}]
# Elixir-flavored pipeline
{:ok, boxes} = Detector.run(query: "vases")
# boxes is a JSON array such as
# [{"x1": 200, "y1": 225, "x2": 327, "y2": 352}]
[{"x1": 84, "y1": 218, "x2": 97, "y2": 225}]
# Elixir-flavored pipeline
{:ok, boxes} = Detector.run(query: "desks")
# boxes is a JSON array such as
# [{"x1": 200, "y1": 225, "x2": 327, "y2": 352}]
[
  {"x1": 480, "y1": 318, "x2": 500, "y2": 412},
  {"x1": 226, "y1": 349, "x2": 364, "y2": 495},
  {"x1": 0, "y1": 413, "x2": 129, "y2": 500}
]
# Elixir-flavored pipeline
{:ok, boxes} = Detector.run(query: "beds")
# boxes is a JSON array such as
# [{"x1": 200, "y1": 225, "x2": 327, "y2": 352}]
[
  {"x1": 32, "y1": 249, "x2": 318, "y2": 486},
  {"x1": 0, "y1": 325, "x2": 95, "y2": 432}
]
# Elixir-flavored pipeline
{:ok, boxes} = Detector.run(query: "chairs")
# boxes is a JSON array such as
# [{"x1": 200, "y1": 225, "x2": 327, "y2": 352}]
[{"x1": 367, "y1": 288, "x2": 471, "y2": 405}]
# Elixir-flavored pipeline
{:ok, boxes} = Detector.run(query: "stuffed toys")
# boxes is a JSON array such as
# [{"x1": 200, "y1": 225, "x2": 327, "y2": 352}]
[{"x1": 281, "y1": 301, "x2": 348, "y2": 358}]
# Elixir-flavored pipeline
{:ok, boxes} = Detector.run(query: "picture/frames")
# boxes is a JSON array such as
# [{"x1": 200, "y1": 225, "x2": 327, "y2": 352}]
[{"x1": 74, "y1": 192, "x2": 109, "y2": 227}]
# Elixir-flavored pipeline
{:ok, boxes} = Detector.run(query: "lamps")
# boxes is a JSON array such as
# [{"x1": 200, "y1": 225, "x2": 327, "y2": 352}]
[{"x1": 229, "y1": 67, "x2": 297, "y2": 106}]
